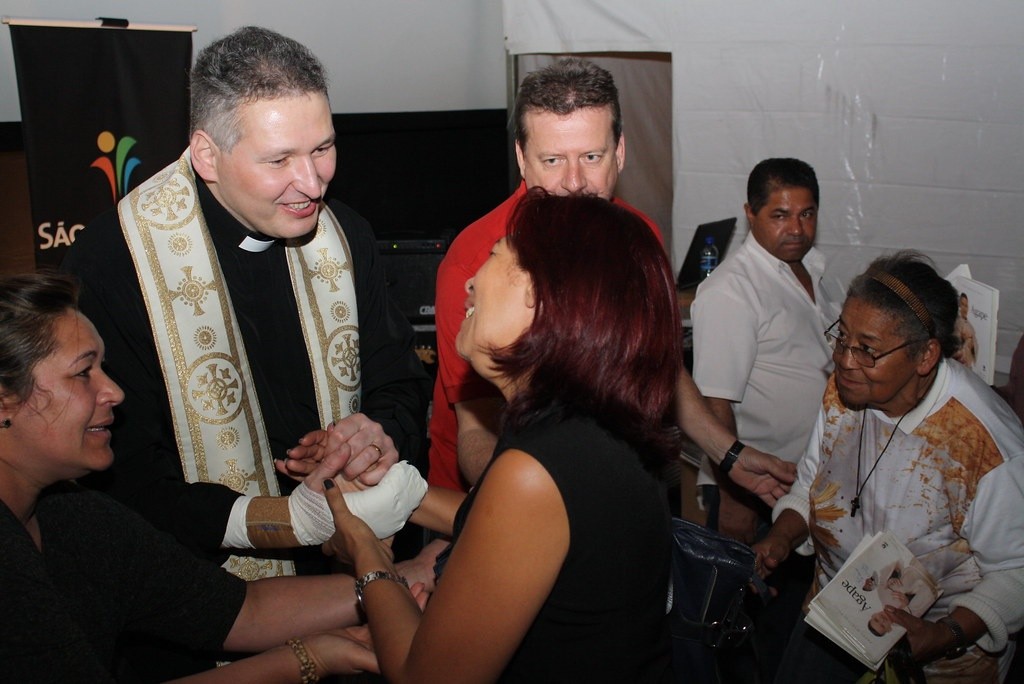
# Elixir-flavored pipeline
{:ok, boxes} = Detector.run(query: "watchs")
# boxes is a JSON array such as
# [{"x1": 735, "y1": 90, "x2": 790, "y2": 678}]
[
  {"x1": 935, "y1": 617, "x2": 967, "y2": 660},
  {"x1": 355, "y1": 572, "x2": 409, "y2": 613}
]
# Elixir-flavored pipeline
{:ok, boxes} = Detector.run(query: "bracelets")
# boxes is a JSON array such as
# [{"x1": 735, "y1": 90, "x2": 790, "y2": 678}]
[
  {"x1": 718, "y1": 440, "x2": 745, "y2": 472},
  {"x1": 287, "y1": 638, "x2": 320, "y2": 683}
]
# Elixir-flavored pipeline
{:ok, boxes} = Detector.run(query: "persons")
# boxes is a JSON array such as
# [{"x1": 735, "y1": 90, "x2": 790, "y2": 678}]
[
  {"x1": 0, "y1": 272, "x2": 450, "y2": 684},
  {"x1": 863, "y1": 557, "x2": 938, "y2": 636},
  {"x1": 990, "y1": 335, "x2": 1024, "y2": 423},
  {"x1": 272, "y1": 187, "x2": 687, "y2": 684},
  {"x1": 951, "y1": 292, "x2": 979, "y2": 369},
  {"x1": 69, "y1": 24, "x2": 434, "y2": 684},
  {"x1": 422, "y1": 55, "x2": 797, "y2": 510},
  {"x1": 686, "y1": 156, "x2": 841, "y2": 684},
  {"x1": 749, "y1": 248, "x2": 1024, "y2": 684}
]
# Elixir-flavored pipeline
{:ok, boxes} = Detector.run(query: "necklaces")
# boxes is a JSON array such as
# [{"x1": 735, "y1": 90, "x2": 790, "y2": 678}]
[{"x1": 850, "y1": 367, "x2": 938, "y2": 518}]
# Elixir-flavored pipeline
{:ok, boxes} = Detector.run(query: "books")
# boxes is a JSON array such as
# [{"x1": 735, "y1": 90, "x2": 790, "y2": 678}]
[
  {"x1": 802, "y1": 532, "x2": 939, "y2": 671},
  {"x1": 939, "y1": 261, "x2": 1001, "y2": 386}
]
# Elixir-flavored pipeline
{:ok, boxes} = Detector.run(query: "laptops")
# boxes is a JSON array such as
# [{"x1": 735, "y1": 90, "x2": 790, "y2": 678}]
[{"x1": 676, "y1": 216, "x2": 736, "y2": 291}]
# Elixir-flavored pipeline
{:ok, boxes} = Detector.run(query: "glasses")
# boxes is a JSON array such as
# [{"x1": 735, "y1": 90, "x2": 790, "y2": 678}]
[{"x1": 823, "y1": 319, "x2": 929, "y2": 368}]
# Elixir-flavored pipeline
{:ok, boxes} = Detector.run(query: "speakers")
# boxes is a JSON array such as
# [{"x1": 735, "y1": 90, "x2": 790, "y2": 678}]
[{"x1": 382, "y1": 237, "x2": 459, "y2": 339}]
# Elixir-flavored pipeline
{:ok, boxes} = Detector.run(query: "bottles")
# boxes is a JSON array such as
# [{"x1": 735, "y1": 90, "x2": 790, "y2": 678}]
[{"x1": 699, "y1": 237, "x2": 719, "y2": 280}]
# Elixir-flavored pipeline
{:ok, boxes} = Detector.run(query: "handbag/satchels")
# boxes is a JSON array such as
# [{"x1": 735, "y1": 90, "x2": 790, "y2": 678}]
[{"x1": 667, "y1": 516, "x2": 765, "y2": 684}]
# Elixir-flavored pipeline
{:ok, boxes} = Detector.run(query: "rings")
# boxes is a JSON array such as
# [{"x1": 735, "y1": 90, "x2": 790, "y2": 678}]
[{"x1": 370, "y1": 444, "x2": 383, "y2": 455}]
